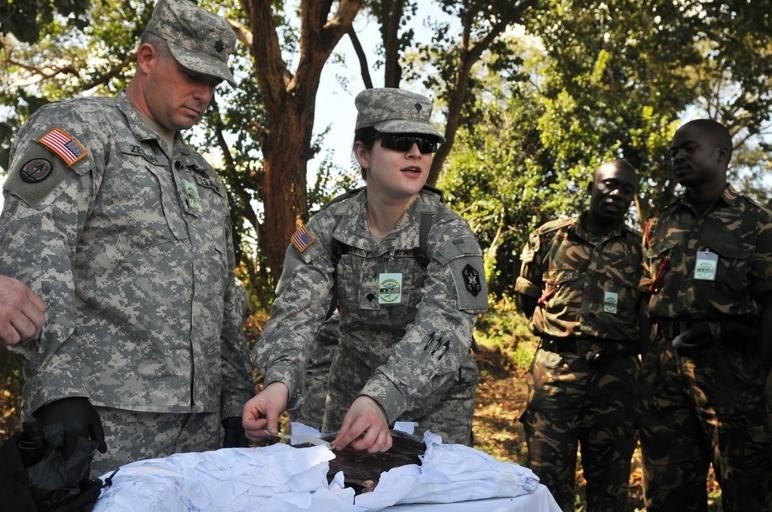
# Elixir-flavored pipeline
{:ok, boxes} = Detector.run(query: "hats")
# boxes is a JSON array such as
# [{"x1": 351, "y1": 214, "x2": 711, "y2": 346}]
[
  {"x1": 354, "y1": 87, "x2": 447, "y2": 144},
  {"x1": 144, "y1": 0, "x2": 238, "y2": 91}
]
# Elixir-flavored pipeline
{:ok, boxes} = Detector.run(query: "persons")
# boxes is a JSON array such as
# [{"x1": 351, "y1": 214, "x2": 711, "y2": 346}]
[
  {"x1": 512, "y1": 157, "x2": 644, "y2": 512},
  {"x1": 0, "y1": 273, "x2": 50, "y2": 347},
  {"x1": 241, "y1": 85, "x2": 490, "y2": 455},
  {"x1": 1, "y1": 0, "x2": 256, "y2": 478},
  {"x1": 631, "y1": 118, "x2": 769, "y2": 512}
]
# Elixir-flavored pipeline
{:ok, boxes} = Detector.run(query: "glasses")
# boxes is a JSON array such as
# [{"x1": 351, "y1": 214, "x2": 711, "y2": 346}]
[{"x1": 365, "y1": 133, "x2": 437, "y2": 155}]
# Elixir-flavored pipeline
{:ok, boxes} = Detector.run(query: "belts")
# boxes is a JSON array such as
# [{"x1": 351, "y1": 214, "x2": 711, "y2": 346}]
[
  {"x1": 654, "y1": 318, "x2": 760, "y2": 343},
  {"x1": 541, "y1": 334, "x2": 638, "y2": 357}
]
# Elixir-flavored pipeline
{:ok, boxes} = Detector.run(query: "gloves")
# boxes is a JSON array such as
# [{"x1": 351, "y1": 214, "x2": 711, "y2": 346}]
[
  {"x1": 221, "y1": 417, "x2": 250, "y2": 448},
  {"x1": 31, "y1": 396, "x2": 107, "y2": 454}
]
